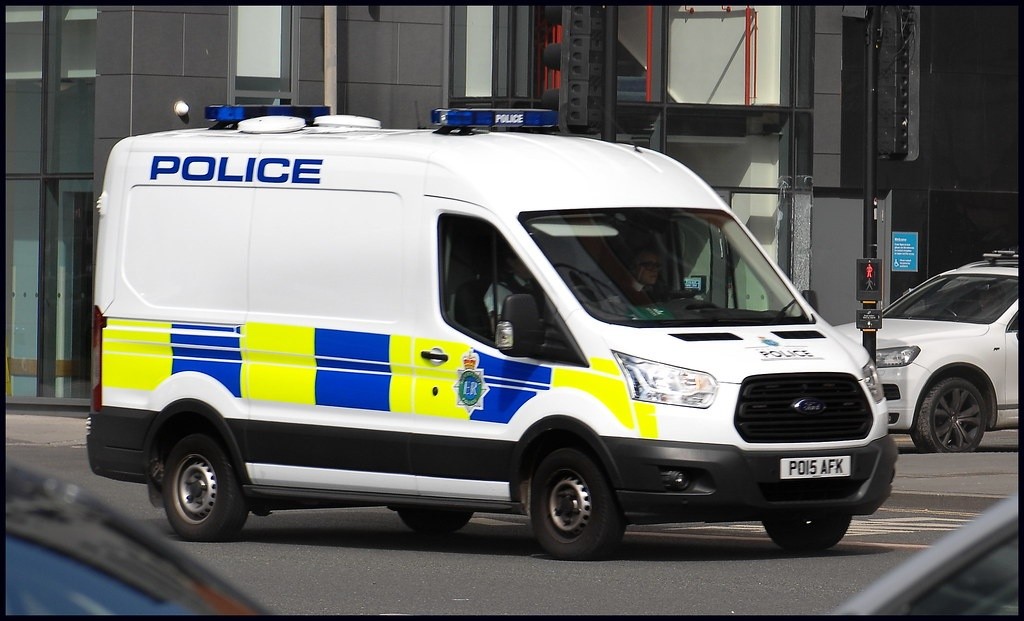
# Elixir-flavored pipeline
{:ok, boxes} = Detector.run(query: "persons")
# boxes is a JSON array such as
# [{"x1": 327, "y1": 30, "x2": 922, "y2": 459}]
[
  {"x1": 613, "y1": 248, "x2": 674, "y2": 308},
  {"x1": 481, "y1": 234, "x2": 554, "y2": 340}
]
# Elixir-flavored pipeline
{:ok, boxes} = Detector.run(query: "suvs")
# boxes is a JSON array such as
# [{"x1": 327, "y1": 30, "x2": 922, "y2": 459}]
[{"x1": 824, "y1": 251, "x2": 1022, "y2": 454}]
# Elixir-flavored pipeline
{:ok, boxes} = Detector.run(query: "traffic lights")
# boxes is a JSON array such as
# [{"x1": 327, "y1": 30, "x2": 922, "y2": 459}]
[{"x1": 856, "y1": 257, "x2": 884, "y2": 304}]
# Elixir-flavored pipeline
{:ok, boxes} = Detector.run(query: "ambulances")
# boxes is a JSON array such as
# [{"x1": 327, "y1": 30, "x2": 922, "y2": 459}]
[{"x1": 85, "y1": 101, "x2": 897, "y2": 563}]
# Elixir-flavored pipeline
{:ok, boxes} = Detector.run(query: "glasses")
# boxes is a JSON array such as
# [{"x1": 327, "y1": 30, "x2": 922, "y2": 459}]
[{"x1": 640, "y1": 261, "x2": 661, "y2": 272}]
